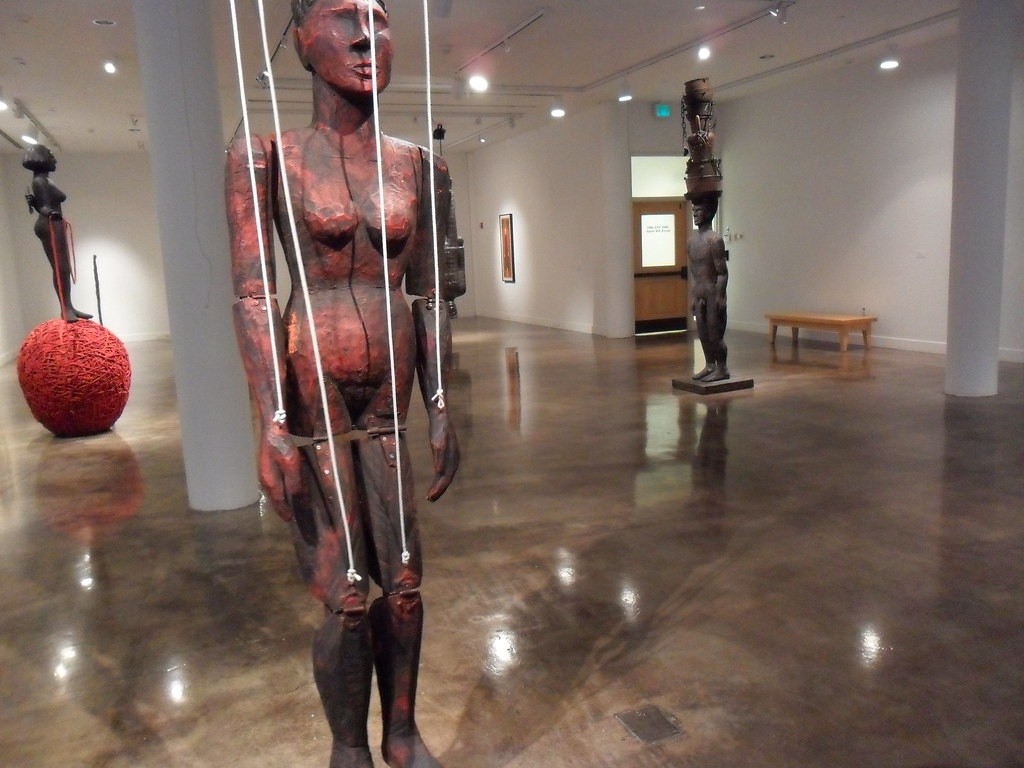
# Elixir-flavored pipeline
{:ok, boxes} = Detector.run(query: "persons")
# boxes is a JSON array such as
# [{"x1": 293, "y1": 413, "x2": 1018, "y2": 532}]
[
  {"x1": 686, "y1": 199, "x2": 731, "y2": 382},
  {"x1": 22, "y1": 144, "x2": 93, "y2": 322},
  {"x1": 226, "y1": 0, "x2": 451, "y2": 768}
]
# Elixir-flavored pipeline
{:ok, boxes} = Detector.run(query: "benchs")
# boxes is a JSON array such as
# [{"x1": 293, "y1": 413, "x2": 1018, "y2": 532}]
[{"x1": 764, "y1": 310, "x2": 879, "y2": 354}]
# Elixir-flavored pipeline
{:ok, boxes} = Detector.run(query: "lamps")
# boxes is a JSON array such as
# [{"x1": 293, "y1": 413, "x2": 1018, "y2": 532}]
[
  {"x1": 503, "y1": 44, "x2": 512, "y2": 54},
  {"x1": 450, "y1": 76, "x2": 465, "y2": 100},
  {"x1": 770, "y1": 9, "x2": 778, "y2": 17},
  {"x1": 21, "y1": 123, "x2": 38, "y2": 144},
  {"x1": 776, "y1": 5, "x2": 785, "y2": 24}
]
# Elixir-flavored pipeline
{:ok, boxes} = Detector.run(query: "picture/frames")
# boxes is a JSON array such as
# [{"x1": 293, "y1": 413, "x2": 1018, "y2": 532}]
[{"x1": 498, "y1": 213, "x2": 515, "y2": 283}]
